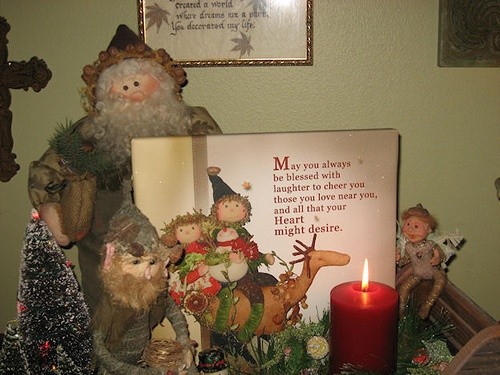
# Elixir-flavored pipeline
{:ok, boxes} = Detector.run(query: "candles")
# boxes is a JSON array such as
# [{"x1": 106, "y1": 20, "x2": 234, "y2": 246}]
[{"x1": 327, "y1": 257, "x2": 398, "y2": 372}]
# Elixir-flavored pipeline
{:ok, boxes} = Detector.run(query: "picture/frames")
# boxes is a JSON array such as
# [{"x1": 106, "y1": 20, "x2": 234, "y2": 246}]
[{"x1": 137, "y1": 0, "x2": 314, "y2": 68}]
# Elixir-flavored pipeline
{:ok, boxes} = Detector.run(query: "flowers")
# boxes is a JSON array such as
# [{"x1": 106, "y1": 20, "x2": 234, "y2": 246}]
[{"x1": 173, "y1": 221, "x2": 455, "y2": 375}]
